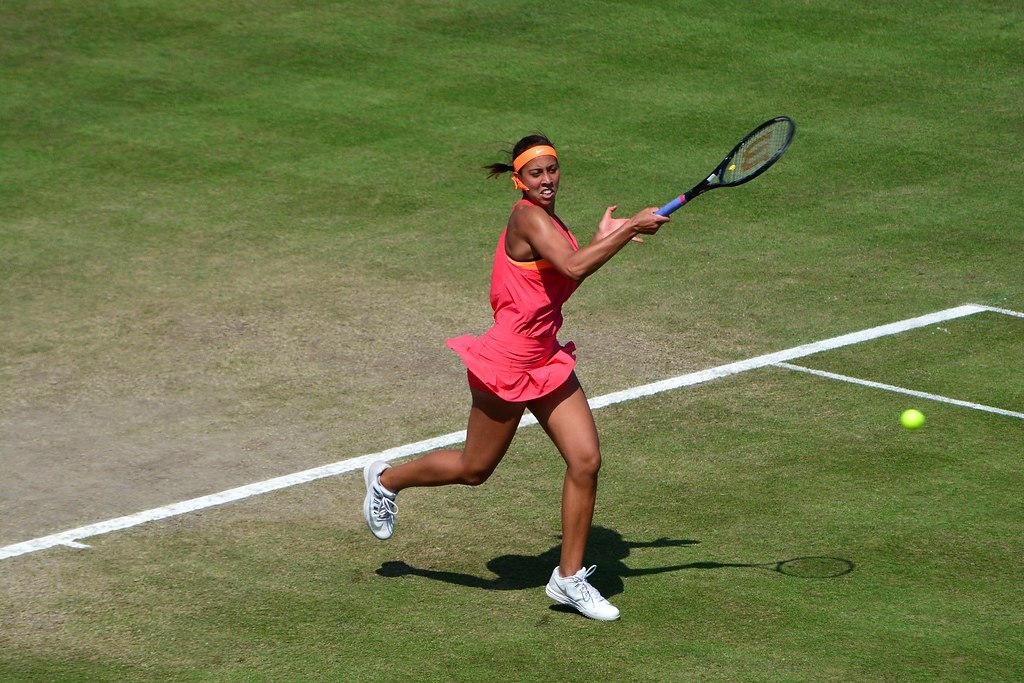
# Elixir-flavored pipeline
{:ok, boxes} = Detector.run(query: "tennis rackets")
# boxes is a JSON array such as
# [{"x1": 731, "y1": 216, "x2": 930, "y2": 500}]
[{"x1": 653, "y1": 115, "x2": 796, "y2": 217}]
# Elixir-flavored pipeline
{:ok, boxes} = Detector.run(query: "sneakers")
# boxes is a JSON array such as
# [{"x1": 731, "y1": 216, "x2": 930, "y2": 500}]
[
  {"x1": 363, "y1": 458, "x2": 399, "y2": 540},
  {"x1": 545, "y1": 565, "x2": 621, "y2": 621}
]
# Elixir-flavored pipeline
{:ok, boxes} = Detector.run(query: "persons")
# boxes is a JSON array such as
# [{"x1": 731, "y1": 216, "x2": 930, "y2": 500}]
[{"x1": 364, "y1": 135, "x2": 669, "y2": 621}]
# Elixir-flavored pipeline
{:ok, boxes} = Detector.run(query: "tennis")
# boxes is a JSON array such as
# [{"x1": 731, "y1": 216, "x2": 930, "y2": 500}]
[{"x1": 899, "y1": 408, "x2": 926, "y2": 431}]
